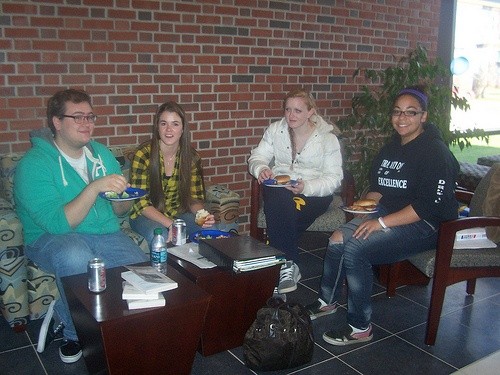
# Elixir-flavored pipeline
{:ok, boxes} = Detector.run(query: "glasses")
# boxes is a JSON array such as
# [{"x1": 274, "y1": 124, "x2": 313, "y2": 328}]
[
  {"x1": 388, "y1": 110, "x2": 425, "y2": 117},
  {"x1": 54, "y1": 113, "x2": 98, "y2": 123}
]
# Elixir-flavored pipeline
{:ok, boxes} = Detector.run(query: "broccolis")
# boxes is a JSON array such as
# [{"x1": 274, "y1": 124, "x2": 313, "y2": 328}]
[{"x1": 105, "y1": 191, "x2": 130, "y2": 199}]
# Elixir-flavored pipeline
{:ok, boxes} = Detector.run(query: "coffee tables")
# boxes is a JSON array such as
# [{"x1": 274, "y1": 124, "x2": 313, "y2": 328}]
[
  {"x1": 61, "y1": 261, "x2": 214, "y2": 375},
  {"x1": 166, "y1": 252, "x2": 285, "y2": 358}
]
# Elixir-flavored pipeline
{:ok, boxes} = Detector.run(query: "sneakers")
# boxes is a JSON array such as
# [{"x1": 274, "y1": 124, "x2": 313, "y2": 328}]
[
  {"x1": 278, "y1": 260, "x2": 301, "y2": 294},
  {"x1": 37, "y1": 300, "x2": 65, "y2": 353},
  {"x1": 322, "y1": 322, "x2": 374, "y2": 346},
  {"x1": 303, "y1": 298, "x2": 338, "y2": 321},
  {"x1": 58, "y1": 338, "x2": 82, "y2": 363},
  {"x1": 272, "y1": 286, "x2": 286, "y2": 303}
]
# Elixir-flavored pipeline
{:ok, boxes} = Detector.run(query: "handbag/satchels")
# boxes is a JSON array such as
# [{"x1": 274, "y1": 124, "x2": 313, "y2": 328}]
[
  {"x1": 189, "y1": 228, "x2": 239, "y2": 243},
  {"x1": 243, "y1": 296, "x2": 315, "y2": 371}
]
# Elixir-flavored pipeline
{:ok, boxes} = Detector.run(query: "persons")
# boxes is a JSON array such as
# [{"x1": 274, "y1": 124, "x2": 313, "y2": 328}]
[
  {"x1": 305, "y1": 86, "x2": 462, "y2": 346},
  {"x1": 12, "y1": 90, "x2": 151, "y2": 364},
  {"x1": 247, "y1": 91, "x2": 344, "y2": 303},
  {"x1": 128, "y1": 101, "x2": 218, "y2": 252}
]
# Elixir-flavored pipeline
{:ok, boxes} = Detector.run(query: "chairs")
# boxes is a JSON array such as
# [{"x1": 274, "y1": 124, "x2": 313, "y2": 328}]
[
  {"x1": 387, "y1": 163, "x2": 500, "y2": 347},
  {"x1": 250, "y1": 168, "x2": 355, "y2": 241}
]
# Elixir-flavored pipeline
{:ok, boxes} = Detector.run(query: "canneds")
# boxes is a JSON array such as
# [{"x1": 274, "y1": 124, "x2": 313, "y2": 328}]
[
  {"x1": 87, "y1": 257, "x2": 106, "y2": 292},
  {"x1": 172, "y1": 218, "x2": 186, "y2": 245}
]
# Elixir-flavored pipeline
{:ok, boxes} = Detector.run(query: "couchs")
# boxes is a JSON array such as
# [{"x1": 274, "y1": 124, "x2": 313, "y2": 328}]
[{"x1": 0, "y1": 144, "x2": 241, "y2": 333}]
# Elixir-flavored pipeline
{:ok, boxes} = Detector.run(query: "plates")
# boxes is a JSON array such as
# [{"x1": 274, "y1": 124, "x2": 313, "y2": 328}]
[
  {"x1": 99, "y1": 187, "x2": 148, "y2": 200},
  {"x1": 342, "y1": 206, "x2": 379, "y2": 213},
  {"x1": 190, "y1": 230, "x2": 234, "y2": 243},
  {"x1": 264, "y1": 180, "x2": 298, "y2": 187}
]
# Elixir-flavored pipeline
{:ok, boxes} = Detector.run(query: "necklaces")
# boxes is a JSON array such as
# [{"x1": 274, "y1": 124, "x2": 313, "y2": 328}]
[{"x1": 163, "y1": 146, "x2": 176, "y2": 165}]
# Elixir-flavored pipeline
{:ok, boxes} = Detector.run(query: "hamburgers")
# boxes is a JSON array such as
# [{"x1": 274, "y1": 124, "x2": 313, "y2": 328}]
[
  {"x1": 273, "y1": 175, "x2": 290, "y2": 185},
  {"x1": 352, "y1": 199, "x2": 377, "y2": 212},
  {"x1": 195, "y1": 209, "x2": 210, "y2": 225}
]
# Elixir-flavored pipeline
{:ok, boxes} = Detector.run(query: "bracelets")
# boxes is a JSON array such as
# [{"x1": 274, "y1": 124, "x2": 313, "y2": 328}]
[
  {"x1": 378, "y1": 217, "x2": 390, "y2": 231},
  {"x1": 168, "y1": 223, "x2": 173, "y2": 230}
]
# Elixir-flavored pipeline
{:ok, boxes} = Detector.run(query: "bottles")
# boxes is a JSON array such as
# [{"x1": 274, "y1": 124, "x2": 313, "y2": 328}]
[{"x1": 151, "y1": 228, "x2": 168, "y2": 277}]
